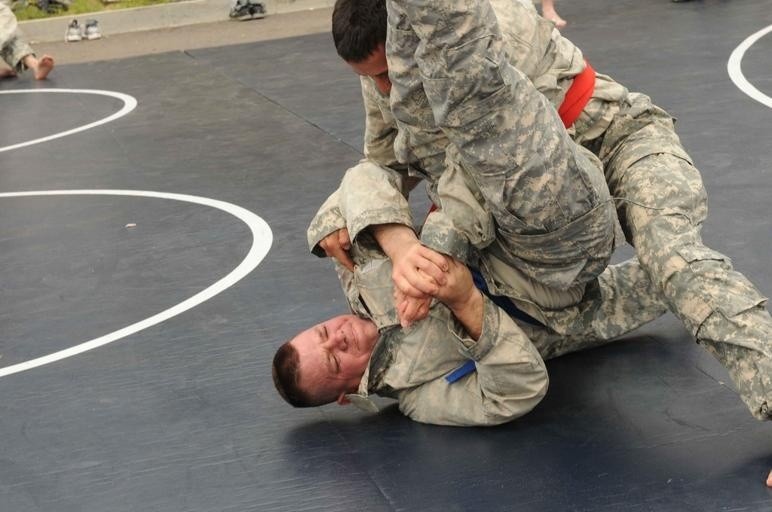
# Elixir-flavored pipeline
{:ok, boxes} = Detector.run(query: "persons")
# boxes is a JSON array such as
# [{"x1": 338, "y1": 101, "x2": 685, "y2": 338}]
[
  {"x1": 272, "y1": 1, "x2": 772, "y2": 488},
  {"x1": 0, "y1": 0, "x2": 54, "y2": 82}
]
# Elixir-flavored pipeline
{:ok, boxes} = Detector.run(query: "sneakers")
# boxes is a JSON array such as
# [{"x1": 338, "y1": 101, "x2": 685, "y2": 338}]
[
  {"x1": 229, "y1": 0, "x2": 264, "y2": 21},
  {"x1": 65, "y1": 18, "x2": 101, "y2": 41}
]
